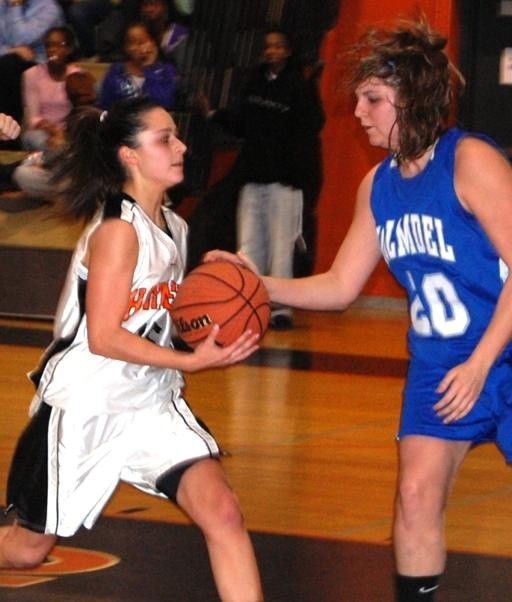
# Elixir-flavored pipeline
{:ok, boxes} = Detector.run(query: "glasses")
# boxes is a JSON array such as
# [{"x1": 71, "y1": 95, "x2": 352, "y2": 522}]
[{"x1": 45, "y1": 40, "x2": 68, "y2": 50}]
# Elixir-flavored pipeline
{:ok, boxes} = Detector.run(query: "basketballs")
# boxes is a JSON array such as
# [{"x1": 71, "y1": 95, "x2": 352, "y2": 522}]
[{"x1": 171, "y1": 260, "x2": 271, "y2": 351}]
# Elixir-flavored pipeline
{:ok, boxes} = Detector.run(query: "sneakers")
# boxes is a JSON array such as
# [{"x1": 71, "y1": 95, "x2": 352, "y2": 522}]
[{"x1": 267, "y1": 313, "x2": 294, "y2": 332}]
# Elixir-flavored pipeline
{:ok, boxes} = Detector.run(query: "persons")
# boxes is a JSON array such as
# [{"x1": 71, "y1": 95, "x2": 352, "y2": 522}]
[
  {"x1": 1, "y1": 0, "x2": 319, "y2": 334},
  {"x1": 0, "y1": 97, "x2": 267, "y2": 602},
  {"x1": 202, "y1": 27, "x2": 511, "y2": 601}
]
